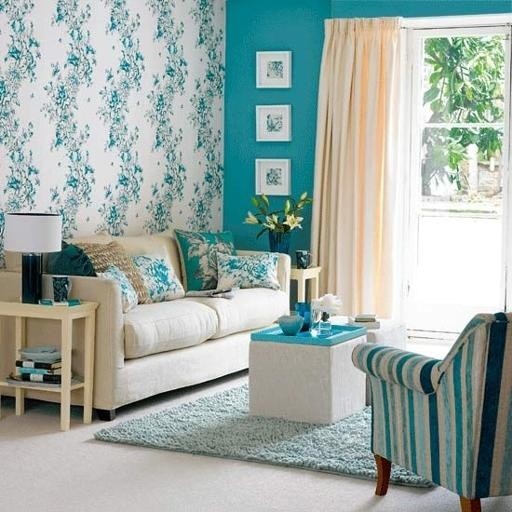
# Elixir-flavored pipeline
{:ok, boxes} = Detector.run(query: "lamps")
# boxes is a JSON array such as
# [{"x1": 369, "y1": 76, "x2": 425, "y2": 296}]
[{"x1": 3, "y1": 211, "x2": 63, "y2": 304}]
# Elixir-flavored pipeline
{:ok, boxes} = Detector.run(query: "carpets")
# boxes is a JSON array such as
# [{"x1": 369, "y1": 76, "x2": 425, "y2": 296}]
[{"x1": 95, "y1": 383, "x2": 433, "y2": 491}]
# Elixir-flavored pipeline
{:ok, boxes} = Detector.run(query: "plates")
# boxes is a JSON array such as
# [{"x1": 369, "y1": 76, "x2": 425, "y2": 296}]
[{"x1": 18, "y1": 346, "x2": 61, "y2": 363}]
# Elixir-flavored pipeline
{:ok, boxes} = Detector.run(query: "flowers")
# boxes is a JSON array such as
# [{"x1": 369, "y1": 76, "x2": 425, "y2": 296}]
[
  {"x1": 243, "y1": 192, "x2": 312, "y2": 240},
  {"x1": 311, "y1": 294, "x2": 341, "y2": 314}
]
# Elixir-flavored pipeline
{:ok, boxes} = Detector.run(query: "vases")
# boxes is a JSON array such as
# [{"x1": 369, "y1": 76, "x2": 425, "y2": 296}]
[
  {"x1": 269, "y1": 231, "x2": 289, "y2": 254},
  {"x1": 318, "y1": 311, "x2": 332, "y2": 335}
]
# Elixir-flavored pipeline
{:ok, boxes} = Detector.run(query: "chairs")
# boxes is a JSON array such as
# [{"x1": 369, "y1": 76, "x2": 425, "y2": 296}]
[{"x1": 351, "y1": 311, "x2": 512, "y2": 512}]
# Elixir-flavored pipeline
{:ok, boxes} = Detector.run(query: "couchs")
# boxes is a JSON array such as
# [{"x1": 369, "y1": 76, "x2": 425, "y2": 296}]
[{"x1": 0, "y1": 233, "x2": 292, "y2": 421}]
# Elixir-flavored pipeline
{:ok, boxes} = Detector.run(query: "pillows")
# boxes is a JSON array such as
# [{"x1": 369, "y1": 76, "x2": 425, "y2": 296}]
[
  {"x1": 80, "y1": 241, "x2": 154, "y2": 305},
  {"x1": 174, "y1": 229, "x2": 237, "y2": 297},
  {"x1": 98, "y1": 265, "x2": 139, "y2": 314},
  {"x1": 133, "y1": 254, "x2": 186, "y2": 302},
  {"x1": 44, "y1": 240, "x2": 97, "y2": 276},
  {"x1": 216, "y1": 251, "x2": 281, "y2": 290}
]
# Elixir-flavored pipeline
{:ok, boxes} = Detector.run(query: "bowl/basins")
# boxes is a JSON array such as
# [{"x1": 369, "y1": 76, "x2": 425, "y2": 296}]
[{"x1": 278, "y1": 316, "x2": 304, "y2": 336}]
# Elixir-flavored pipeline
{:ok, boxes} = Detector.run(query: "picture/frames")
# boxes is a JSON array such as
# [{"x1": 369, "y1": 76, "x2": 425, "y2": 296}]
[
  {"x1": 254, "y1": 103, "x2": 292, "y2": 144},
  {"x1": 255, "y1": 49, "x2": 292, "y2": 90},
  {"x1": 255, "y1": 157, "x2": 291, "y2": 197}
]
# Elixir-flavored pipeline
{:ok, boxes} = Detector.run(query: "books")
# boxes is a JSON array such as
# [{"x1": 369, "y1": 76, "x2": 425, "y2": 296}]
[
  {"x1": 16, "y1": 360, "x2": 73, "y2": 384},
  {"x1": 349, "y1": 310, "x2": 380, "y2": 331}
]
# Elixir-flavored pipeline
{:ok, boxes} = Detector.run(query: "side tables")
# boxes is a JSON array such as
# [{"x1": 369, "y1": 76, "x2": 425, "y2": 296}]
[
  {"x1": 0, "y1": 299, "x2": 100, "y2": 433},
  {"x1": 292, "y1": 264, "x2": 322, "y2": 303}
]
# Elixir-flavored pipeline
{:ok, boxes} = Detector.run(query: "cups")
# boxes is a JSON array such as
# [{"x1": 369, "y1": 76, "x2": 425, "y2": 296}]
[
  {"x1": 287, "y1": 310, "x2": 300, "y2": 317},
  {"x1": 295, "y1": 249, "x2": 314, "y2": 270},
  {"x1": 51, "y1": 276, "x2": 72, "y2": 302}
]
